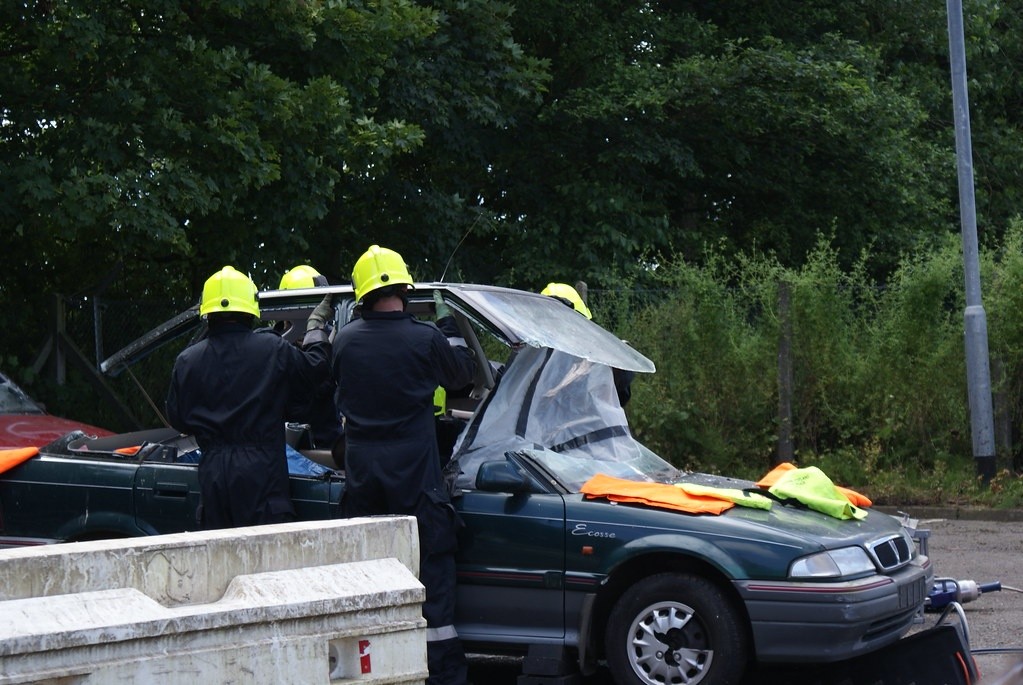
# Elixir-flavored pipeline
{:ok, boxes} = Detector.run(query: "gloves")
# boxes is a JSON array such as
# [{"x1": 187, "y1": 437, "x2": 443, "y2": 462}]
[
  {"x1": 306, "y1": 292, "x2": 335, "y2": 333},
  {"x1": 433, "y1": 289, "x2": 455, "y2": 320}
]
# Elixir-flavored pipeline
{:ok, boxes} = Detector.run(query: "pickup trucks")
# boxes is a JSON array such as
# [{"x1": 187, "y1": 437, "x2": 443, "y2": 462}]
[{"x1": 0, "y1": 282, "x2": 934, "y2": 685}]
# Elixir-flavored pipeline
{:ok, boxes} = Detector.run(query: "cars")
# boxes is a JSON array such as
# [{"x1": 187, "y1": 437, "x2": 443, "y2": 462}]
[{"x1": 0, "y1": 370, "x2": 118, "y2": 456}]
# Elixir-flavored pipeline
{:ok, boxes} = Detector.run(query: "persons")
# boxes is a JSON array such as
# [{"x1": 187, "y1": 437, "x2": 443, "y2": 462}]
[
  {"x1": 531, "y1": 281, "x2": 631, "y2": 414},
  {"x1": 324, "y1": 244, "x2": 478, "y2": 684},
  {"x1": 269, "y1": 262, "x2": 346, "y2": 459},
  {"x1": 165, "y1": 262, "x2": 339, "y2": 543}
]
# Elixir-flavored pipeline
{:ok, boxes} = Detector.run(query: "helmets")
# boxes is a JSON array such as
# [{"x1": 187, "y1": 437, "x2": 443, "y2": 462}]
[
  {"x1": 200, "y1": 266, "x2": 261, "y2": 319},
  {"x1": 279, "y1": 265, "x2": 330, "y2": 289},
  {"x1": 350, "y1": 245, "x2": 415, "y2": 304},
  {"x1": 540, "y1": 283, "x2": 593, "y2": 322}
]
